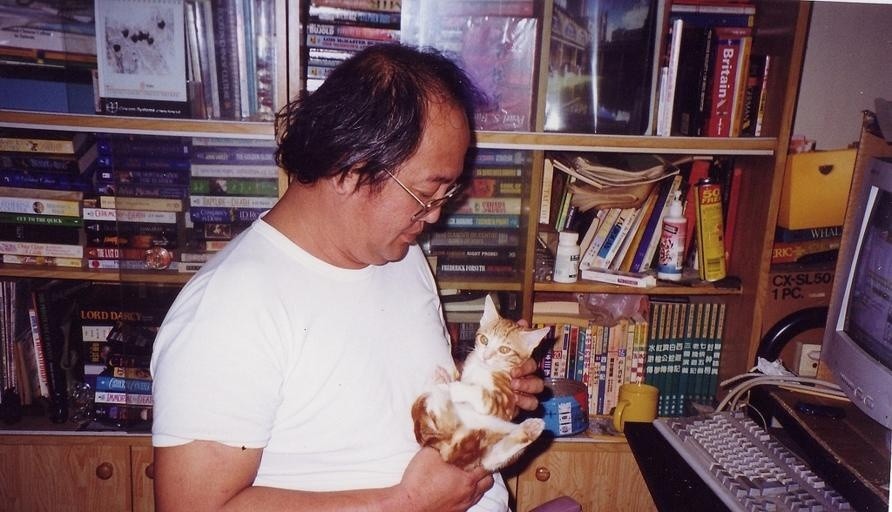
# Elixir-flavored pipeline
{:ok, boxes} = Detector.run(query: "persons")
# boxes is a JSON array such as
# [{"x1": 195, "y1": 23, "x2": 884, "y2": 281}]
[{"x1": 150, "y1": 45, "x2": 544, "y2": 512}]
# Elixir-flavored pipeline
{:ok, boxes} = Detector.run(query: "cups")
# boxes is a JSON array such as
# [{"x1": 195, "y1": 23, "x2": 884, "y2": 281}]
[{"x1": 613, "y1": 382, "x2": 659, "y2": 430}]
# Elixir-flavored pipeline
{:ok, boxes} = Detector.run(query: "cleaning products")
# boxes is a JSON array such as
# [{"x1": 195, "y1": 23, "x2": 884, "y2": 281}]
[
  {"x1": 658, "y1": 189, "x2": 687, "y2": 281},
  {"x1": 693, "y1": 177, "x2": 726, "y2": 281}
]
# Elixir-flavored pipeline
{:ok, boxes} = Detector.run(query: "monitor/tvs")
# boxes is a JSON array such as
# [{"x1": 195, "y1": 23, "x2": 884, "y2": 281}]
[{"x1": 823, "y1": 155, "x2": 892, "y2": 432}]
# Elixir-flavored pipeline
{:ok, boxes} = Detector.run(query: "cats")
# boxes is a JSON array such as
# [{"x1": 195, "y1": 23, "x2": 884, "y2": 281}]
[{"x1": 411, "y1": 294, "x2": 551, "y2": 472}]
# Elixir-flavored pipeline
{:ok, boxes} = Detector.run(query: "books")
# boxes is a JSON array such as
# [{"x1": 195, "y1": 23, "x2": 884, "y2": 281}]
[
  {"x1": 1, "y1": 280, "x2": 180, "y2": 431},
  {"x1": 3, "y1": 2, "x2": 276, "y2": 122},
  {"x1": 301, "y1": 2, "x2": 539, "y2": 130},
  {"x1": 440, "y1": 289, "x2": 524, "y2": 363},
  {"x1": 547, "y1": 1, "x2": 771, "y2": 139},
  {"x1": 536, "y1": 150, "x2": 745, "y2": 287},
  {"x1": 3, "y1": 126, "x2": 280, "y2": 274},
  {"x1": 530, "y1": 292, "x2": 726, "y2": 416},
  {"x1": 412, "y1": 148, "x2": 524, "y2": 277}
]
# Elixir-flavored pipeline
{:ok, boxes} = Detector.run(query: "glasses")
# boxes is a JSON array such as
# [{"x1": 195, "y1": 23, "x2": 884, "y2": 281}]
[{"x1": 385, "y1": 169, "x2": 463, "y2": 222}]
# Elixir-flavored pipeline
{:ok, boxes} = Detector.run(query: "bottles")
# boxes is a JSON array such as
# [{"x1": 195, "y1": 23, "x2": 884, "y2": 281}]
[
  {"x1": 657, "y1": 176, "x2": 728, "y2": 283},
  {"x1": 554, "y1": 229, "x2": 582, "y2": 284}
]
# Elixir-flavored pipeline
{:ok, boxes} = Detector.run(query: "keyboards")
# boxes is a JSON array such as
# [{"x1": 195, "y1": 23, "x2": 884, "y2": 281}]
[{"x1": 653, "y1": 409, "x2": 857, "y2": 512}]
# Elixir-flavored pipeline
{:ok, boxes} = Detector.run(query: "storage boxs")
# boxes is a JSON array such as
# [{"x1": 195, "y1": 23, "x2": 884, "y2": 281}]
[{"x1": 761, "y1": 147, "x2": 859, "y2": 378}]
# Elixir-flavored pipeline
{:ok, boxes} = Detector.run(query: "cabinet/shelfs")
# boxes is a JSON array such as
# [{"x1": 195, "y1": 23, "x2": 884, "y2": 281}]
[
  {"x1": 623, "y1": 382, "x2": 890, "y2": 512},
  {"x1": 0, "y1": 0, "x2": 817, "y2": 511}
]
